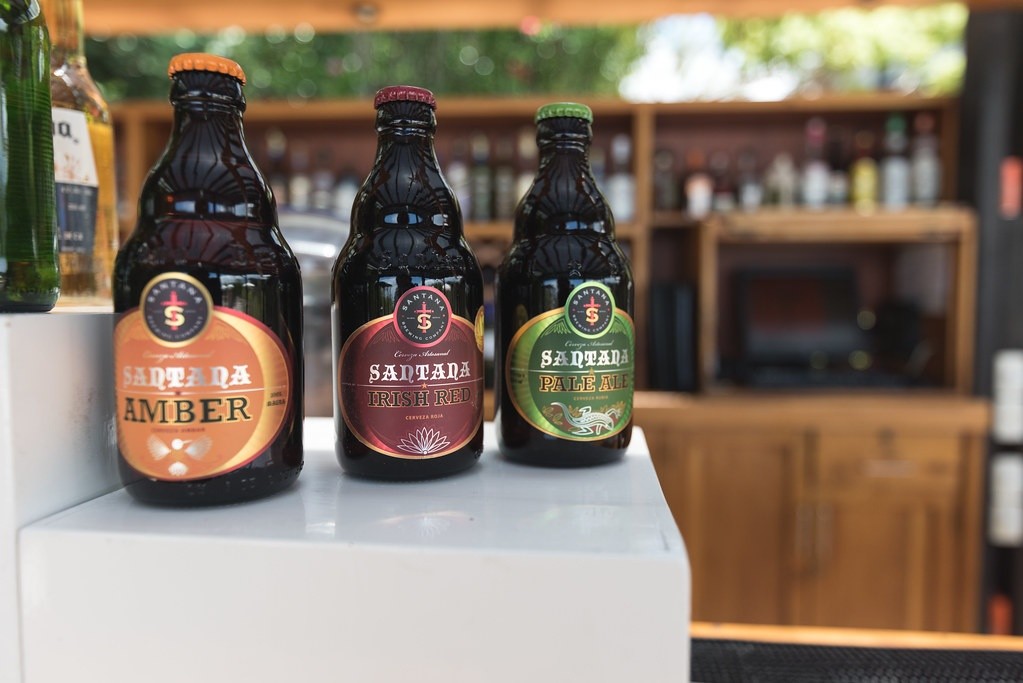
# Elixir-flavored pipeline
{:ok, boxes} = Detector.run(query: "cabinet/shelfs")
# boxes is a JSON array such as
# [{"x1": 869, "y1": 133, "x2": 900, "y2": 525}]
[{"x1": 106, "y1": 98, "x2": 990, "y2": 640}]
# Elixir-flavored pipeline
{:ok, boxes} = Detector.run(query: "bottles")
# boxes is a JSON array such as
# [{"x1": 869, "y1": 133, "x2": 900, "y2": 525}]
[
  {"x1": 1, "y1": 0, "x2": 60, "y2": 313},
  {"x1": 657, "y1": 115, "x2": 948, "y2": 217},
  {"x1": 112, "y1": 55, "x2": 637, "y2": 510},
  {"x1": 40, "y1": 1, "x2": 119, "y2": 307}
]
